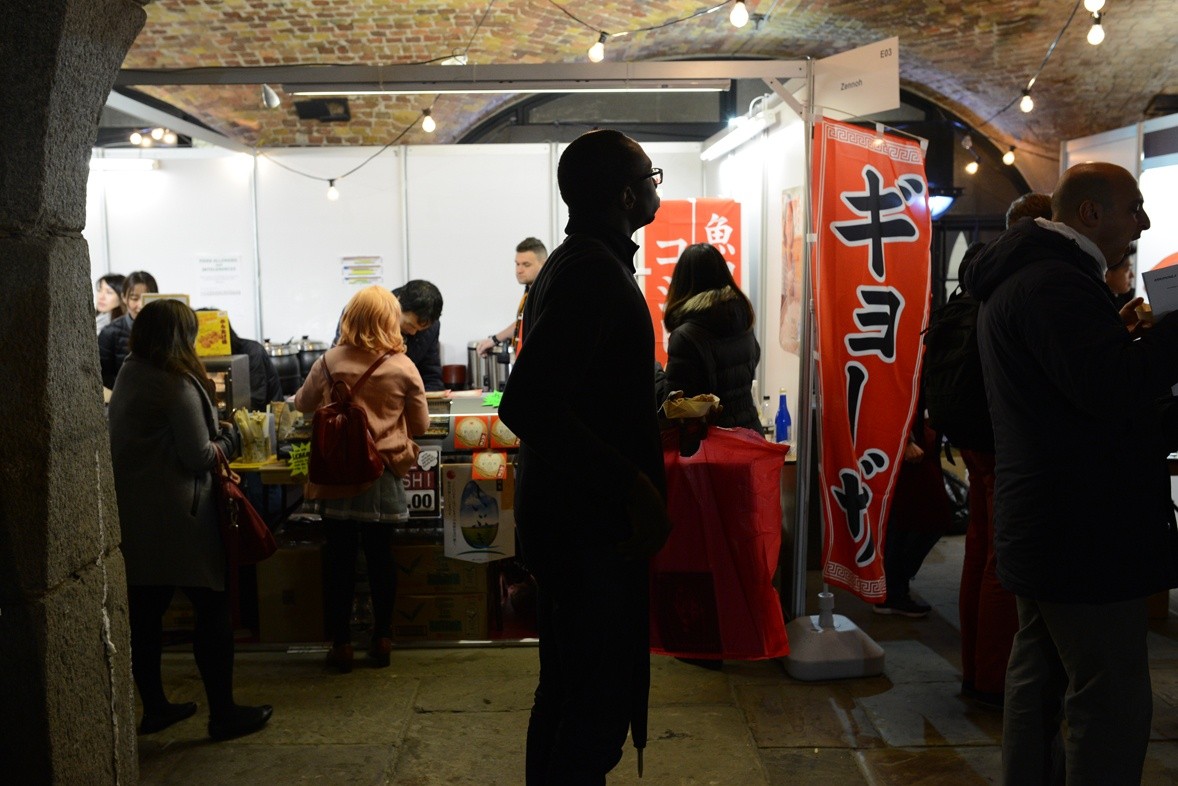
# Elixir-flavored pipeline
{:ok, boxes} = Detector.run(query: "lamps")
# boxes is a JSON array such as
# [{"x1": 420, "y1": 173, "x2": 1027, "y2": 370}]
[
  {"x1": 280, "y1": 80, "x2": 731, "y2": 93},
  {"x1": 700, "y1": 110, "x2": 777, "y2": 162},
  {"x1": 264, "y1": 83, "x2": 281, "y2": 110}
]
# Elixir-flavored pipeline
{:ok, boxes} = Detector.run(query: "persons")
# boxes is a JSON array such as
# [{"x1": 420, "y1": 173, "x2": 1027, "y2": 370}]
[
  {"x1": 111, "y1": 300, "x2": 274, "y2": 744},
  {"x1": 969, "y1": 161, "x2": 1178, "y2": 786},
  {"x1": 475, "y1": 238, "x2": 548, "y2": 356},
  {"x1": 1106, "y1": 240, "x2": 1135, "y2": 308},
  {"x1": 94, "y1": 272, "x2": 126, "y2": 337},
  {"x1": 199, "y1": 306, "x2": 288, "y2": 417},
  {"x1": 659, "y1": 236, "x2": 768, "y2": 439},
  {"x1": 927, "y1": 190, "x2": 1056, "y2": 705},
  {"x1": 496, "y1": 129, "x2": 723, "y2": 784},
  {"x1": 293, "y1": 284, "x2": 431, "y2": 666},
  {"x1": 98, "y1": 272, "x2": 159, "y2": 403},
  {"x1": 331, "y1": 280, "x2": 444, "y2": 393}
]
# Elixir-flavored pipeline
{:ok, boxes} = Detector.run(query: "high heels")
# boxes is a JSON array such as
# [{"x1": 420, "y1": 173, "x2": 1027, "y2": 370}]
[
  {"x1": 365, "y1": 637, "x2": 390, "y2": 668},
  {"x1": 324, "y1": 645, "x2": 354, "y2": 672}
]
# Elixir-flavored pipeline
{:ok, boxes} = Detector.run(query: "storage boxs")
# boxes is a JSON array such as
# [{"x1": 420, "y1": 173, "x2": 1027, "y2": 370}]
[
  {"x1": 490, "y1": 416, "x2": 521, "y2": 448},
  {"x1": 254, "y1": 541, "x2": 491, "y2": 643},
  {"x1": 455, "y1": 415, "x2": 490, "y2": 449}
]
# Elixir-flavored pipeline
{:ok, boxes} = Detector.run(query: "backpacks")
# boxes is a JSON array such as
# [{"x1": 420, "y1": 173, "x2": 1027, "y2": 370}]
[
  {"x1": 919, "y1": 284, "x2": 992, "y2": 451},
  {"x1": 307, "y1": 351, "x2": 397, "y2": 485}
]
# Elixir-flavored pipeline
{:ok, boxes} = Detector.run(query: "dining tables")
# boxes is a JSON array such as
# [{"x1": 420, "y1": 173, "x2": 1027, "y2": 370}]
[{"x1": 231, "y1": 451, "x2": 522, "y2": 630}]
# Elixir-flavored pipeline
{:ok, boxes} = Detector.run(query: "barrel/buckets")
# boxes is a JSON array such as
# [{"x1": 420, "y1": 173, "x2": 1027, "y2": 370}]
[
  {"x1": 294, "y1": 337, "x2": 327, "y2": 388},
  {"x1": 467, "y1": 341, "x2": 511, "y2": 394},
  {"x1": 266, "y1": 340, "x2": 302, "y2": 396}
]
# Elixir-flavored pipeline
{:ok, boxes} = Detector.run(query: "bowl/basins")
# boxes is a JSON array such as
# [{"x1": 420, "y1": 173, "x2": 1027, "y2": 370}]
[{"x1": 664, "y1": 389, "x2": 722, "y2": 433}]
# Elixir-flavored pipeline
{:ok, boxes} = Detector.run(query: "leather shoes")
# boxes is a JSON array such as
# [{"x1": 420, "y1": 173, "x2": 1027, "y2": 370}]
[
  {"x1": 207, "y1": 705, "x2": 272, "y2": 740},
  {"x1": 142, "y1": 702, "x2": 197, "y2": 731}
]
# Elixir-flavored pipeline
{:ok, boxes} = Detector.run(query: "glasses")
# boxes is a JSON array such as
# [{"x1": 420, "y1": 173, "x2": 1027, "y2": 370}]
[{"x1": 631, "y1": 168, "x2": 663, "y2": 188}]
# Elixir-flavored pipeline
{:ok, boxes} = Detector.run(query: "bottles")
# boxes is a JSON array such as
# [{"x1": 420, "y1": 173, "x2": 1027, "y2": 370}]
[
  {"x1": 777, "y1": 388, "x2": 793, "y2": 446},
  {"x1": 761, "y1": 393, "x2": 776, "y2": 445}
]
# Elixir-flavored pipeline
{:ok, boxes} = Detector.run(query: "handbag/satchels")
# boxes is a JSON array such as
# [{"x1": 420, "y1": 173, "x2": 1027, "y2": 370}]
[{"x1": 211, "y1": 442, "x2": 278, "y2": 566}]
